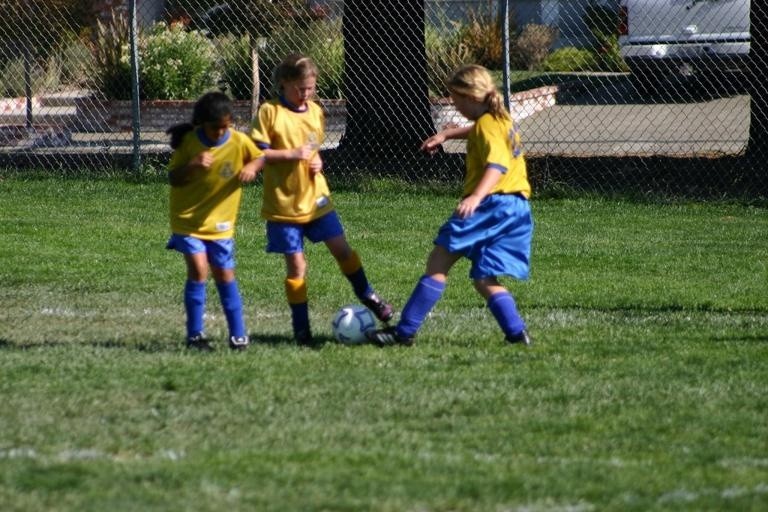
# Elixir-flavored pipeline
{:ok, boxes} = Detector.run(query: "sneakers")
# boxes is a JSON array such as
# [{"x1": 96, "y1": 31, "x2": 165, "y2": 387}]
[
  {"x1": 504, "y1": 330, "x2": 530, "y2": 345},
  {"x1": 229, "y1": 335, "x2": 249, "y2": 350},
  {"x1": 187, "y1": 331, "x2": 215, "y2": 353},
  {"x1": 295, "y1": 329, "x2": 314, "y2": 348},
  {"x1": 361, "y1": 290, "x2": 393, "y2": 321},
  {"x1": 364, "y1": 326, "x2": 416, "y2": 348}
]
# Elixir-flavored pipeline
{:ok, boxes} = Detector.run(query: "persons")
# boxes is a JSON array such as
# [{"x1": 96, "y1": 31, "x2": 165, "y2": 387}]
[
  {"x1": 251, "y1": 53, "x2": 396, "y2": 346},
  {"x1": 366, "y1": 64, "x2": 536, "y2": 346},
  {"x1": 167, "y1": 92, "x2": 267, "y2": 353}
]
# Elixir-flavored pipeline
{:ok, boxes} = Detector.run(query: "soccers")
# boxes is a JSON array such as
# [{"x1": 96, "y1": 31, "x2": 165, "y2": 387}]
[{"x1": 332, "y1": 303, "x2": 376, "y2": 345}]
